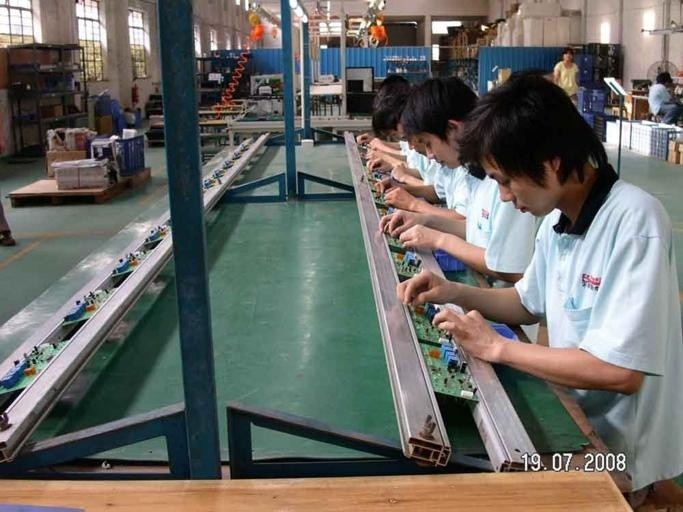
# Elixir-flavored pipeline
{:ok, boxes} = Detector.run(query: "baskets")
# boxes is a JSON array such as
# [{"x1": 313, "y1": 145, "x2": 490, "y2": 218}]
[
  {"x1": 581, "y1": 112, "x2": 681, "y2": 162},
  {"x1": 576, "y1": 88, "x2": 607, "y2": 113},
  {"x1": 110, "y1": 100, "x2": 141, "y2": 132},
  {"x1": 41, "y1": 103, "x2": 63, "y2": 118},
  {"x1": 89, "y1": 134, "x2": 145, "y2": 177},
  {"x1": 88, "y1": 95, "x2": 111, "y2": 116}
]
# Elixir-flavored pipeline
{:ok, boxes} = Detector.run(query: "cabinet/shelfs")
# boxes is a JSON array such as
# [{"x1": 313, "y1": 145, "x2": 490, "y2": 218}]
[{"x1": 8, "y1": 42, "x2": 91, "y2": 158}]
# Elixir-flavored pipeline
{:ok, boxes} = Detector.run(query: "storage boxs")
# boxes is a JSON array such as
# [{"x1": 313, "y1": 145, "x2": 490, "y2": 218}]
[
  {"x1": 34, "y1": 131, "x2": 145, "y2": 190},
  {"x1": 92, "y1": 114, "x2": 113, "y2": 137},
  {"x1": 572, "y1": 85, "x2": 683, "y2": 162}
]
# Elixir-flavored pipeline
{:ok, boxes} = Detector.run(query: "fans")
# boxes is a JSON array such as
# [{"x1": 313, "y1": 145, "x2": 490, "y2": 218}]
[{"x1": 645, "y1": 60, "x2": 678, "y2": 87}]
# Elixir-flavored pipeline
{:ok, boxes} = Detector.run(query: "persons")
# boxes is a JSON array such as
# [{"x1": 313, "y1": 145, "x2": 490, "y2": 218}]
[
  {"x1": 355, "y1": 74, "x2": 440, "y2": 199},
  {"x1": 0, "y1": 201, "x2": 15, "y2": 245},
  {"x1": 377, "y1": 75, "x2": 548, "y2": 345},
  {"x1": 553, "y1": 47, "x2": 579, "y2": 109},
  {"x1": 397, "y1": 71, "x2": 683, "y2": 506},
  {"x1": 383, "y1": 76, "x2": 485, "y2": 221},
  {"x1": 649, "y1": 72, "x2": 678, "y2": 123}
]
